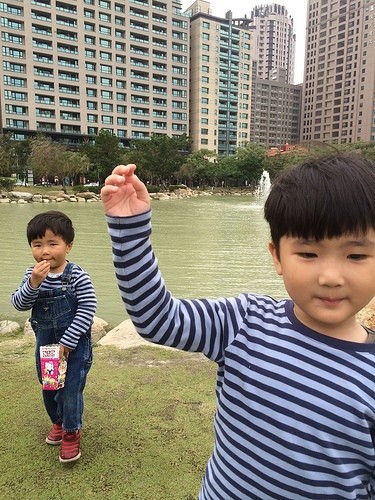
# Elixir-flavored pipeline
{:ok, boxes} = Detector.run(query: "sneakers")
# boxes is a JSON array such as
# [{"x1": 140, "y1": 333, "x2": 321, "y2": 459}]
[
  {"x1": 45, "y1": 419, "x2": 64, "y2": 445},
  {"x1": 58, "y1": 425, "x2": 82, "y2": 463}
]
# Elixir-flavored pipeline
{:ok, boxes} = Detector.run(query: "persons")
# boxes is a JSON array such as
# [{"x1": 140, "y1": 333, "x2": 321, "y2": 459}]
[
  {"x1": 12, "y1": 211, "x2": 98, "y2": 462},
  {"x1": 100, "y1": 141, "x2": 374, "y2": 499}
]
